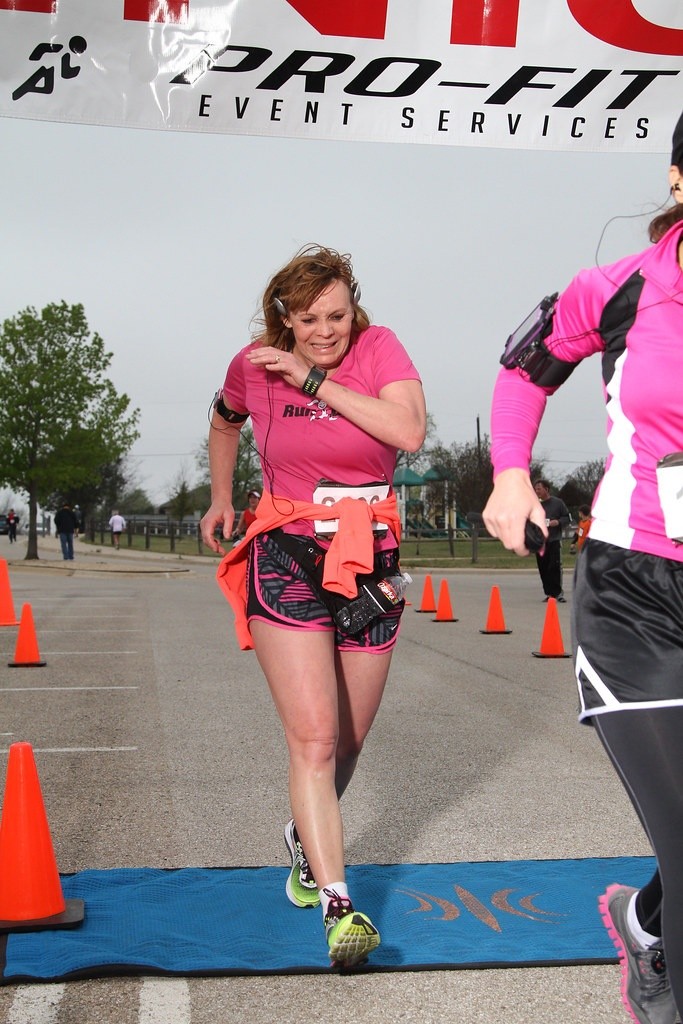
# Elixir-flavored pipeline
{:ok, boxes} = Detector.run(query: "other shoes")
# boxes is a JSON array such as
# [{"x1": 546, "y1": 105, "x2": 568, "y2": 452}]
[
  {"x1": 115, "y1": 545, "x2": 119, "y2": 550},
  {"x1": 543, "y1": 595, "x2": 552, "y2": 603},
  {"x1": 556, "y1": 595, "x2": 566, "y2": 602}
]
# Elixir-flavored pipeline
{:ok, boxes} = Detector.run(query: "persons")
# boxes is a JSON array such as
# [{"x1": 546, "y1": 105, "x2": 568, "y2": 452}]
[
  {"x1": 5, "y1": 509, "x2": 20, "y2": 544},
  {"x1": 531, "y1": 480, "x2": 572, "y2": 603},
  {"x1": 482, "y1": 113, "x2": 683, "y2": 1024},
  {"x1": 109, "y1": 512, "x2": 126, "y2": 551},
  {"x1": 200, "y1": 245, "x2": 428, "y2": 972},
  {"x1": 72, "y1": 505, "x2": 82, "y2": 537},
  {"x1": 571, "y1": 504, "x2": 592, "y2": 554},
  {"x1": 231, "y1": 489, "x2": 260, "y2": 537},
  {"x1": 54, "y1": 500, "x2": 80, "y2": 560}
]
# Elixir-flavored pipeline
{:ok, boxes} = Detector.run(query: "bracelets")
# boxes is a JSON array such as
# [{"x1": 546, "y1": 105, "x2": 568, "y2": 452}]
[{"x1": 302, "y1": 364, "x2": 327, "y2": 396}]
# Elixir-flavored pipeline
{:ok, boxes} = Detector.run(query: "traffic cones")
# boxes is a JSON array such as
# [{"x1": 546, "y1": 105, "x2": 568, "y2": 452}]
[
  {"x1": 8, "y1": 603, "x2": 46, "y2": 667},
  {"x1": 431, "y1": 578, "x2": 459, "y2": 622},
  {"x1": 0, "y1": 556, "x2": 21, "y2": 626},
  {"x1": 415, "y1": 575, "x2": 437, "y2": 613},
  {"x1": 0, "y1": 742, "x2": 84, "y2": 931},
  {"x1": 478, "y1": 586, "x2": 513, "y2": 634},
  {"x1": 532, "y1": 596, "x2": 573, "y2": 658}
]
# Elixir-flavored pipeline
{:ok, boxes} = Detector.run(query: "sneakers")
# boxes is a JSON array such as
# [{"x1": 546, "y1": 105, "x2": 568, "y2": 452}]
[
  {"x1": 321, "y1": 887, "x2": 379, "y2": 972},
  {"x1": 599, "y1": 882, "x2": 676, "y2": 1024},
  {"x1": 284, "y1": 819, "x2": 321, "y2": 908}
]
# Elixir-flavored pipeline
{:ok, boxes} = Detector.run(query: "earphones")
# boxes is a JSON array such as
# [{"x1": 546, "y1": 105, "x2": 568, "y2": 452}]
[{"x1": 672, "y1": 183, "x2": 680, "y2": 190}]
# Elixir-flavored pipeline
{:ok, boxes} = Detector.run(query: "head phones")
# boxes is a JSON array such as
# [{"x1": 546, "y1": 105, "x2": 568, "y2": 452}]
[{"x1": 274, "y1": 282, "x2": 362, "y2": 316}]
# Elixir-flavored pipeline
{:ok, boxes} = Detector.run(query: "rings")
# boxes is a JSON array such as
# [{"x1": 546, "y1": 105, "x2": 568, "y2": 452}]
[{"x1": 275, "y1": 354, "x2": 280, "y2": 364}]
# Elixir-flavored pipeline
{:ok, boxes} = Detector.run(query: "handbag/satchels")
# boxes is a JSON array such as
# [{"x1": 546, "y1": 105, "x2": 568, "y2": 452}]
[{"x1": 265, "y1": 525, "x2": 403, "y2": 636}]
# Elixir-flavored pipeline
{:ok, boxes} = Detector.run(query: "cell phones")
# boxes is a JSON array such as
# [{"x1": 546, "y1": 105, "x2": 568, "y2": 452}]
[{"x1": 503, "y1": 302, "x2": 546, "y2": 364}]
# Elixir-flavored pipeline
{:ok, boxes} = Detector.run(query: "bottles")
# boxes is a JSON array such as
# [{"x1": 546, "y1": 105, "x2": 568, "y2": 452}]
[{"x1": 333, "y1": 573, "x2": 413, "y2": 636}]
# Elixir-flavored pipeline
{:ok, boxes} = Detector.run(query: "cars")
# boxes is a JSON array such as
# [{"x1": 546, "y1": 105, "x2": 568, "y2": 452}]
[{"x1": 26, "y1": 523, "x2": 48, "y2": 535}]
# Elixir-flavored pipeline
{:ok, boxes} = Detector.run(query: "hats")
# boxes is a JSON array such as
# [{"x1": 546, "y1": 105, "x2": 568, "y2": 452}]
[
  {"x1": 246, "y1": 490, "x2": 261, "y2": 500},
  {"x1": 75, "y1": 505, "x2": 79, "y2": 509}
]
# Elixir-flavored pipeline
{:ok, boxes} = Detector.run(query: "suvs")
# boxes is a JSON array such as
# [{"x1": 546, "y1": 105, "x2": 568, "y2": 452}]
[{"x1": 0, "y1": 515, "x2": 8, "y2": 534}]
